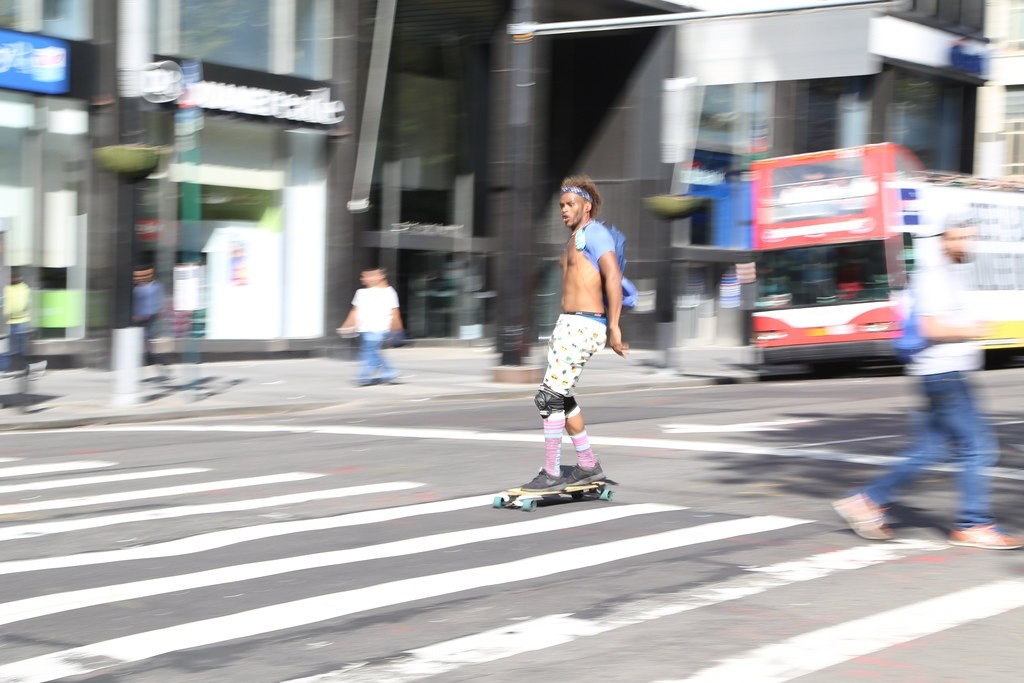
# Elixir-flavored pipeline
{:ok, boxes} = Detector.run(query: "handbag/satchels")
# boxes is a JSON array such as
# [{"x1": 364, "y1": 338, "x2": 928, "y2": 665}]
[{"x1": 894, "y1": 311, "x2": 928, "y2": 350}]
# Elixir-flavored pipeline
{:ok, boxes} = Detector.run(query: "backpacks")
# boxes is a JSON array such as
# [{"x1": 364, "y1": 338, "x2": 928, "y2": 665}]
[{"x1": 575, "y1": 220, "x2": 639, "y2": 310}]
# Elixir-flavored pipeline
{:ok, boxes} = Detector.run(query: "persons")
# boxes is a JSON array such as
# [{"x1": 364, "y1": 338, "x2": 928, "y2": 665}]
[
  {"x1": 516, "y1": 174, "x2": 632, "y2": 492},
  {"x1": 828, "y1": 211, "x2": 1024, "y2": 551},
  {"x1": 337, "y1": 268, "x2": 405, "y2": 388}
]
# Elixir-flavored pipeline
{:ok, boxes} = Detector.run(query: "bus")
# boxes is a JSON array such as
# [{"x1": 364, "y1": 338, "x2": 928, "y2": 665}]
[{"x1": 746, "y1": 141, "x2": 1022, "y2": 379}]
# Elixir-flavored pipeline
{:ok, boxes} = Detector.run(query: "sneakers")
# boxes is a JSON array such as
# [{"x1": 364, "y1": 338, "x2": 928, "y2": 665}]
[
  {"x1": 565, "y1": 462, "x2": 604, "y2": 486},
  {"x1": 832, "y1": 493, "x2": 895, "y2": 539},
  {"x1": 521, "y1": 468, "x2": 566, "y2": 491},
  {"x1": 950, "y1": 522, "x2": 1023, "y2": 549}
]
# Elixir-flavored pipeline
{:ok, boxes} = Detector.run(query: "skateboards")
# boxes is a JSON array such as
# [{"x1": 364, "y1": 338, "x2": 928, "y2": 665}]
[{"x1": 494, "y1": 481, "x2": 612, "y2": 511}]
